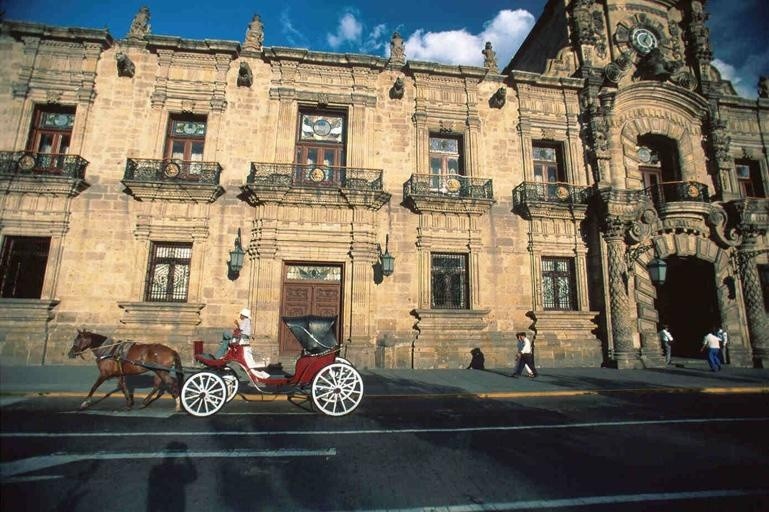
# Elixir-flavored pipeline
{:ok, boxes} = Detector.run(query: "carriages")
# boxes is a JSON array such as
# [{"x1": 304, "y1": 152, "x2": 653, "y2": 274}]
[{"x1": 68, "y1": 315, "x2": 364, "y2": 417}]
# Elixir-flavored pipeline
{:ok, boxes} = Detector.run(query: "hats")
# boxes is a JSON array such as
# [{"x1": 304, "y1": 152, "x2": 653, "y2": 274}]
[{"x1": 239, "y1": 309, "x2": 251, "y2": 319}]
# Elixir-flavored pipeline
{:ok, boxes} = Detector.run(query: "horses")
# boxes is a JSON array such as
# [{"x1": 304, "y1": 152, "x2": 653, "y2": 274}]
[{"x1": 67, "y1": 328, "x2": 185, "y2": 413}]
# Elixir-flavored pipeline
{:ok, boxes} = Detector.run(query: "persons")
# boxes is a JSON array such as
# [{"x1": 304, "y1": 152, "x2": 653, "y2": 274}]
[
  {"x1": 715, "y1": 325, "x2": 728, "y2": 364},
  {"x1": 214, "y1": 308, "x2": 251, "y2": 359},
  {"x1": 658, "y1": 324, "x2": 673, "y2": 364},
  {"x1": 700, "y1": 328, "x2": 722, "y2": 371},
  {"x1": 511, "y1": 331, "x2": 538, "y2": 379}
]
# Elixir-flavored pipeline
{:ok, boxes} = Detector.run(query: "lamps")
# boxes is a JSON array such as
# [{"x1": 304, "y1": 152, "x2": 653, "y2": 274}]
[
  {"x1": 380, "y1": 233, "x2": 395, "y2": 278},
  {"x1": 229, "y1": 229, "x2": 245, "y2": 275}
]
